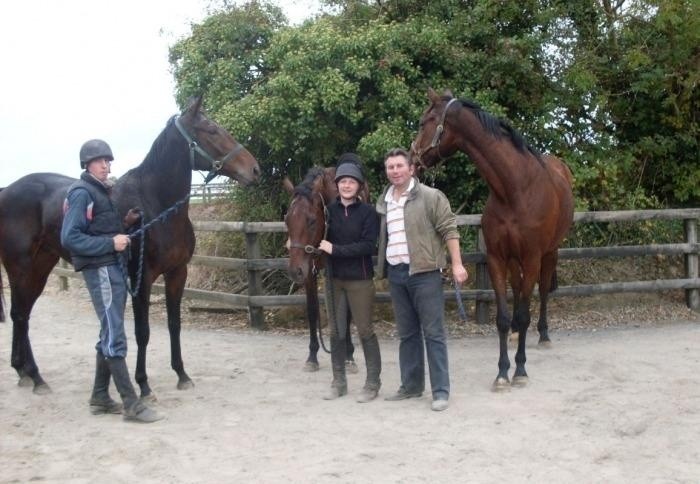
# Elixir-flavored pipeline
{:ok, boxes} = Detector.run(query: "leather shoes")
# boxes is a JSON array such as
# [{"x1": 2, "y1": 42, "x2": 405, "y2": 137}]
[
  {"x1": 385, "y1": 386, "x2": 422, "y2": 401},
  {"x1": 432, "y1": 399, "x2": 448, "y2": 410},
  {"x1": 357, "y1": 384, "x2": 379, "y2": 403},
  {"x1": 324, "y1": 384, "x2": 347, "y2": 399}
]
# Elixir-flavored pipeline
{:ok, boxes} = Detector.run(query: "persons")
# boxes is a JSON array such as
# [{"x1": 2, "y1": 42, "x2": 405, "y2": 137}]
[
  {"x1": 60, "y1": 138, "x2": 163, "y2": 422},
  {"x1": 286, "y1": 162, "x2": 382, "y2": 403},
  {"x1": 376, "y1": 147, "x2": 469, "y2": 411}
]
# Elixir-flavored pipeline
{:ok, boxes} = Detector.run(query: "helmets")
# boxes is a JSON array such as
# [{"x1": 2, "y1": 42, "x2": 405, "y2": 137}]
[
  {"x1": 334, "y1": 162, "x2": 364, "y2": 183},
  {"x1": 80, "y1": 140, "x2": 113, "y2": 168}
]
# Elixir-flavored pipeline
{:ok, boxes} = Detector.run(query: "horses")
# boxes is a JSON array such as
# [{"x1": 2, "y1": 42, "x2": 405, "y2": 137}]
[
  {"x1": 408, "y1": 85, "x2": 576, "y2": 391},
  {"x1": 280, "y1": 166, "x2": 372, "y2": 375},
  {"x1": 0, "y1": 90, "x2": 263, "y2": 403}
]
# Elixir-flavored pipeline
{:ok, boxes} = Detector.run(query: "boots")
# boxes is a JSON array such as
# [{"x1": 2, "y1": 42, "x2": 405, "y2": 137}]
[{"x1": 91, "y1": 352, "x2": 164, "y2": 422}]
